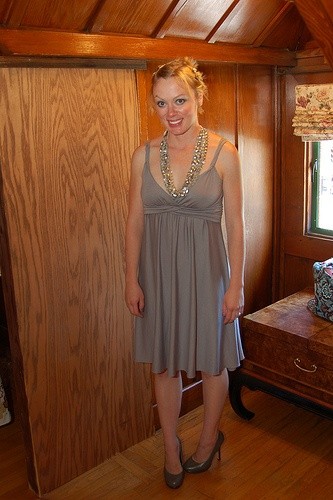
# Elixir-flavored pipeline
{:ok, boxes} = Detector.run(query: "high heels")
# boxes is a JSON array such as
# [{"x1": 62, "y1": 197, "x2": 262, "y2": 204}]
[
  {"x1": 183, "y1": 430, "x2": 224, "y2": 473},
  {"x1": 164, "y1": 435, "x2": 184, "y2": 489}
]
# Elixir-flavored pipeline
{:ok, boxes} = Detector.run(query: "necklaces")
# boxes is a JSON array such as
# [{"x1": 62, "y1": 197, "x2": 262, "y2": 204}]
[{"x1": 159, "y1": 128, "x2": 208, "y2": 197}]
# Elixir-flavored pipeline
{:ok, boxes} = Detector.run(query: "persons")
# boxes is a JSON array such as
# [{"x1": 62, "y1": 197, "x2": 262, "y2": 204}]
[{"x1": 124, "y1": 56, "x2": 245, "y2": 488}]
